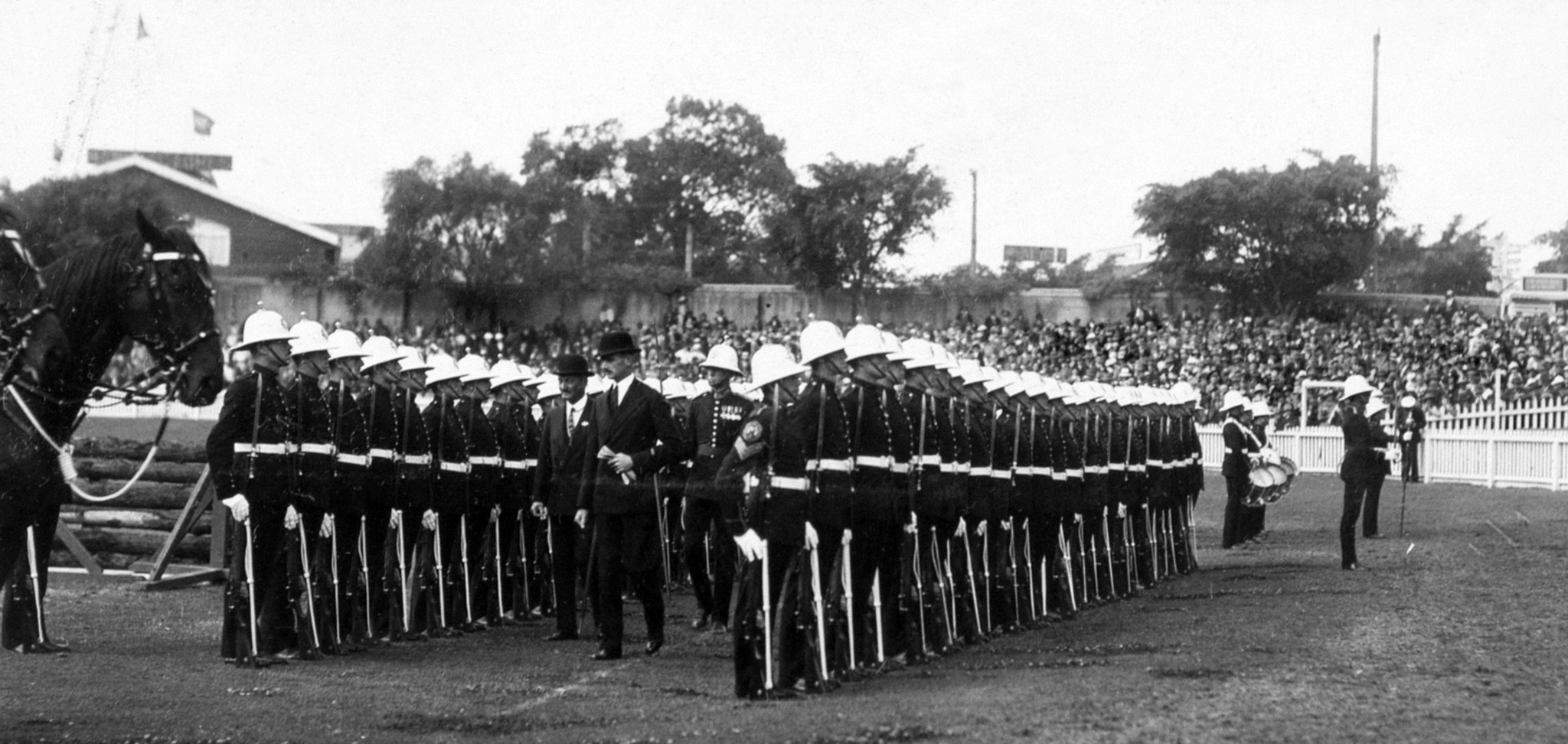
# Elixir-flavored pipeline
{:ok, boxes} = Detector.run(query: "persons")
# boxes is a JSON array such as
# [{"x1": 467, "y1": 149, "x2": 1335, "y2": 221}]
[
  {"x1": 206, "y1": 310, "x2": 687, "y2": 672},
  {"x1": 573, "y1": 333, "x2": 679, "y2": 660},
  {"x1": 1338, "y1": 375, "x2": 1414, "y2": 572},
  {"x1": 679, "y1": 319, "x2": 1205, "y2": 701},
  {"x1": 1395, "y1": 391, "x2": 1426, "y2": 484},
  {"x1": 96, "y1": 287, "x2": 1568, "y2": 431},
  {"x1": 529, "y1": 354, "x2": 600, "y2": 641},
  {"x1": 1219, "y1": 390, "x2": 1275, "y2": 551}
]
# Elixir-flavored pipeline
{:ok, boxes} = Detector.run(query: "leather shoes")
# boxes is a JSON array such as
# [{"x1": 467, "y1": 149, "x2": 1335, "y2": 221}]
[
  {"x1": 1366, "y1": 532, "x2": 1384, "y2": 538},
  {"x1": 711, "y1": 619, "x2": 726, "y2": 635},
  {"x1": 590, "y1": 649, "x2": 623, "y2": 661},
  {"x1": 1343, "y1": 561, "x2": 1371, "y2": 570},
  {"x1": 646, "y1": 637, "x2": 662, "y2": 654},
  {"x1": 693, "y1": 609, "x2": 711, "y2": 629},
  {"x1": 542, "y1": 631, "x2": 577, "y2": 640}
]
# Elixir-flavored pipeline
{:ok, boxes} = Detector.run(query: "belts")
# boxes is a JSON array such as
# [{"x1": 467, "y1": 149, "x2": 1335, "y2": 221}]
[
  {"x1": 235, "y1": 442, "x2": 540, "y2": 474},
  {"x1": 1225, "y1": 449, "x2": 1249, "y2": 454},
  {"x1": 750, "y1": 452, "x2": 1202, "y2": 490}
]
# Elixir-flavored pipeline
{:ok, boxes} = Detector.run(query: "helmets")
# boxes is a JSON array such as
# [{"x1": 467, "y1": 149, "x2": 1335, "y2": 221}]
[
  {"x1": 1218, "y1": 391, "x2": 1272, "y2": 417},
  {"x1": 1367, "y1": 401, "x2": 1389, "y2": 417},
  {"x1": 1338, "y1": 374, "x2": 1378, "y2": 402},
  {"x1": 230, "y1": 308, "x2": 759, "y2": 400},
  {"x1": 748, "y1": 320, "x2": 1202, "y2": 406}
]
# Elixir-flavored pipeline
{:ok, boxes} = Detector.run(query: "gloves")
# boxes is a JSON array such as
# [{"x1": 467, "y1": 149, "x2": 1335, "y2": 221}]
[
  {"x1": 223, "y1": 493, "x2": 438, "y2": 537},
  {"x1": 800, "y1": 515, "x2": 817, "y2": 549},
  {"x1": 734, "y1": 527, "x2": 765, "y2": 563}
]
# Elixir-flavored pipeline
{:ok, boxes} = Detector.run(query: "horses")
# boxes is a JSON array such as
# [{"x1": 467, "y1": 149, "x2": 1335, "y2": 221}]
[
  {"x1": 0, "y1": 209, "x2": 67, "y2": 404},
  {"x1": 0, "y1": 208, "x2": 224, "y2": 659}
]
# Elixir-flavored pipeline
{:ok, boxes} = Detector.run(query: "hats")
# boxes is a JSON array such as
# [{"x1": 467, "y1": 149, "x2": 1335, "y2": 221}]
[
  {"x1": 594, "y1": 332, "x2": 639, "y2": 356},
  {"x1": 549, "y1": 355, "x2": 594, "y2": 377}
]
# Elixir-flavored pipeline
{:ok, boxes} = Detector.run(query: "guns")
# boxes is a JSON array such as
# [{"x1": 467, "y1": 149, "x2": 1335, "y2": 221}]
[
  {"x1": 314, "y1": 380, "x2": 345, "y2": 657},
  {"x1": 227, "y1": 372, "x2": 262, "y2": 670},
  {"x1": 822, "y1": 386, "x2": 858, "y2": 683},
  {"x1": 482, "y1": 395, "x2": 511, "y2": 628},
  {"x1": 382, "y1": 388, "x2": 408, "y2": 645},
  {"x1": 285, "y1": 374, "x2": 324, "y2": 660},
  {"x1": 453, "y1": 393, "x2": 475, "y2": 632},
  {"x1": 795, "y1": 382, "x2": 830, "y2": 695},
  {"x1": 510, "y1": 397, "x2": 557, "y2": 620},
  {"x1": 929, "y1": 396, "x2": 1199, "y2": 652},
  {"x1": 899, "y1": 393, "x2": 927, "y2": 666},
  {"x1": 349, "y1": 384, "x2": 373, "y2": 649},
  {"x1": 742, "y1": 383, "x2": 776, "y2": 702},
  {"x1": 422, "y1": 390, "x2": 446, "y2": 639}
]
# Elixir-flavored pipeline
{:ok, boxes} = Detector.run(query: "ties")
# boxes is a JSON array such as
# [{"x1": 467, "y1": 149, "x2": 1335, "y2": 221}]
[{"x1": 568, "y1": 408, "x2": 574, "y2": 434}]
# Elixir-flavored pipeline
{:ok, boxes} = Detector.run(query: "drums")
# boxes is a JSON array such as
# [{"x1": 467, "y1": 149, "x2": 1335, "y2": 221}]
[
  {"x1": 1240, "y1": 466, "x2": 1274, "y2": 508},
  {"x1": 1280, "y1": 456, "x2": 1295, "y2": 495},
  {"x1": 1266, "y1": 464, "x2": 1287, "y2": 502}
]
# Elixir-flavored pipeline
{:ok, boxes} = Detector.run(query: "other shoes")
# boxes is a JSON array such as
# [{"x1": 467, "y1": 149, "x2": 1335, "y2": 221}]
[
  {"x1": 228, "y1": 610, "x2": 534, "y2": 662},
  {"x1": 749, "y1": 531, "x2": 1271, "y2": 700}
]
